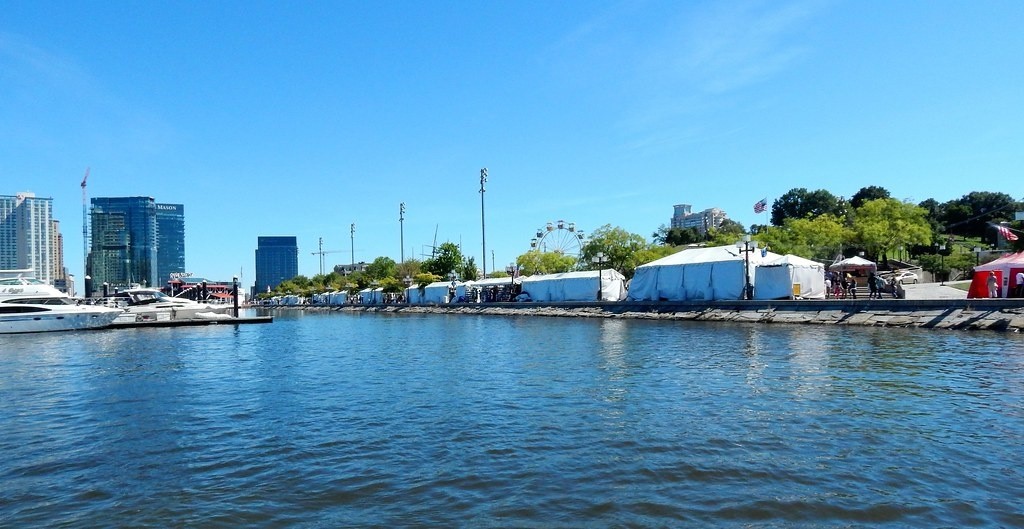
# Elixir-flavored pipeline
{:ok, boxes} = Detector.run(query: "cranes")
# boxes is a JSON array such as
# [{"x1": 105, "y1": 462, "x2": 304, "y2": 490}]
[{"x1": 80, "y1": 166, "x2": 91, "y2": 276}]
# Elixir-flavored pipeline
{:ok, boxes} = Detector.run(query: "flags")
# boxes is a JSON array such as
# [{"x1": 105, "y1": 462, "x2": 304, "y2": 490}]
[
  {"x1": 991, "y1": 225, "x2": 1018, "y2": 240},
  {"x1": 753, "y1": 197, "x2": 767, "y2": 214}
]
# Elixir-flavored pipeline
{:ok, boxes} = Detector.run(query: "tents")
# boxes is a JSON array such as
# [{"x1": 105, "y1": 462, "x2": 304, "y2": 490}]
[
  {"x1": 967, "y1": 251, "x2": 1024, "y2": 298},
  {"x1": 521, "y1": 268, "x2": 627, "y2": 301},
  {"x1": 829, "y1": 255, "x2": 877, "y2": 276},
  {"x1": 404, "y1": 280, "x2": 461, "y2": 304},
  {"x1": 626, "y1": 244, "x2": 782, "y2": 300},
  {"x1": 270, "y1": 286, "x2": 395, "y2": 306},
  {"x1": 754, "y1": 253, "x2": 825, "y2": 300},
  {"x1": 455, "y1": 275, "x2": 528, "y2": 303}
]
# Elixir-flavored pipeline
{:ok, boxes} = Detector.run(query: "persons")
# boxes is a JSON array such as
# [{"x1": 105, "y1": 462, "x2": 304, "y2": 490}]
[
  {"x1": 986, "y1": 271, "x2": 998, "y2": 297},
  {"x1": 890, "y1": 279, "x2": 899, "y2": 298},
  {"x1": 833, "y1": 276, "x2": 857, "y2": 299},
  {"x1": 472, "y1": 285, "x2": 520, "y2": 302},
  {"x1": 824, "y1": 278, "x2": 832, "y2": 299},
  {"x1": 1016, "y1": 272, "x2": 1024, "y2": 298},
  {"x1": 868, "y1": 272, "x2": 884, "y2": 299}
]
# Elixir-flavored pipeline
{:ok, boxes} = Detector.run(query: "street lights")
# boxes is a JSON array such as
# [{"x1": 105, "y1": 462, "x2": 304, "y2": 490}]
[
  {"x1": 325, "y1": 285, "x2": 333, "y2": 304},
  {"x1": 591, "y1": 251, "x2": 611, "y2": 302},
  {"x1": 939, "y1": 242, "x2": 947, "y2": 286},
  {"x1": 398, "y1": 201, "x2": 406, "y2": 266},
  {"x1": 403, "y1": 273, "x2": 413, "y2": 305},
  {"x1": 350, "y1": 222, "x2": 357, "y2": 275},
  {"x1": 343, "y1": 282, "x2": 353, "y2": 305},
  {"x1": 318, "y1": 236, "x2": 323, "y2": 276},
  {"x1": 972, "y1": 247, "x2": 982, "y2": 267},
  {"x1": 732, "y1": 233, "x2": 758, "y2": 300},
  {"x1": 448, "y1": 269, "x2": 458, "y2": 300},
  {"x1": 505, "y1": 262, "x2": 518, "y2": 301},
  {"x1": 480, "y1": 167, "x2": 489, "y2": 279},
  {"x1": 369, "y1": 279, "x2": 379, "y2": 302}
]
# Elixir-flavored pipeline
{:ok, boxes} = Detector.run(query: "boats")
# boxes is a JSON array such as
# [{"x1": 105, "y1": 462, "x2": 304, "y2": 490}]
[{"x1": 0, "y1": 275, "x2": 235, "y2": 333}]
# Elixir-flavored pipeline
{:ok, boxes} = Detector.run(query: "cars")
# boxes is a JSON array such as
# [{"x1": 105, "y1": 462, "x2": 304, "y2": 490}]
[{"x1": 887, "y1": 271, "x2": 918, "y2": 286}]
